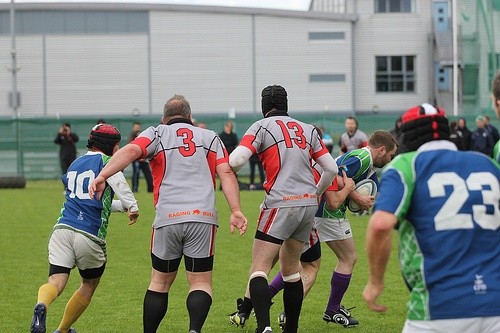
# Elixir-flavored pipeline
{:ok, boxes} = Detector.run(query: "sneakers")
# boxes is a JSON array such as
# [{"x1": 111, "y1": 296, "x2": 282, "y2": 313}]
[
  {"x1": 229, "y1": 312, "x2": 246, "y2": 328},
  {"x1": 255, "y1": 326, "x2": 272, "y2": 333},
  {"x1": 30, "y1": 303, "x2": 46, "y2": 333},
  {"x1": 277, "y1": 312, "x2": 286, "y2": 331},
  {"x1": 322, "y1": 305, "x2": 360, "y2": 328}
]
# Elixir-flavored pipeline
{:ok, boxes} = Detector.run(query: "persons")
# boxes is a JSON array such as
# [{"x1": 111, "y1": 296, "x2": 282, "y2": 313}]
[
  {"x1": 389, "y1": 117, "x2": 404, "y2": 158},
  {"x1": 457, "y1": 118, "x2": 472, "y2": 151},
  {"x1": 248, "y1": 153, "x2": 265, "y2": 191},
  {"x1": 227, "y1": 84, "x2": 338, "y2": 333},
  {"x1": 87, "y1": 94, "x2": 248, "y2": 333},
  {"x1": 319, "y1": 127, "x2": 333, "y2": 155},
  {"x1": 482, "y1": 114, "x2": 500, "y2": 145},
  {"x1": 339, "y1": 117, "x2": 380, "y2": 192},
  {"x1": 251, "y1": 130, "x2": 398, "y2": 327},
  {"x1": 448, "y1": 121, "x2": 466, "y2": 151},
  {"x1": 55, "y1": 125, "x2": 79, "y2": 197},
  {"x1": 127, "y1": 122, "x2": 154, "y2": 192},
  {"x1": 219, "y1": 120, "x2": 239, "y2": 154},
  {"x1": 491, "y1": 71, "x2": 500, "y2": 164},
  {"x1": 226, "y1": 126, "x2": 356, "y2": 333},
  {"x1": 361, "y1": 103, "x2": 500, "y2": 333},
  {"x1": 470, "y1": 115, "x2": 493, "y2": 157}
]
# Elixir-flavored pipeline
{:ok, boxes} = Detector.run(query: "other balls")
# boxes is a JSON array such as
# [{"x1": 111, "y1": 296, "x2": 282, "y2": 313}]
[{"x1": 347, "y1": 178, "x2": 377, "y2": 213}]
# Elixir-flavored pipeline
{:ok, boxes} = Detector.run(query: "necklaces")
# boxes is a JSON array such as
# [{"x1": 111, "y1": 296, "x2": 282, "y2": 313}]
[{"x1": 31, "y1": 125, "x2": 140, "y2": 333}]
